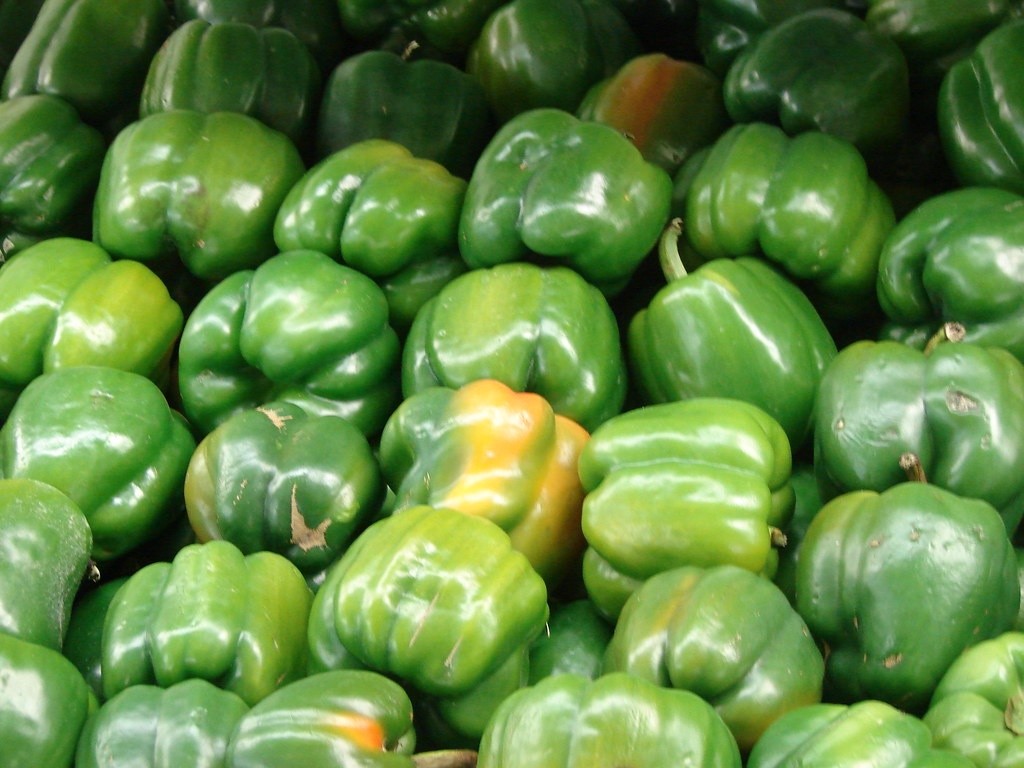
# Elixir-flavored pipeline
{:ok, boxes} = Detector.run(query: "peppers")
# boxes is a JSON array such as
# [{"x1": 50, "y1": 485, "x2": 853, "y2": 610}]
[{"x1": 0, "y1": 0, "x2": 1024, "y2": 768}]
